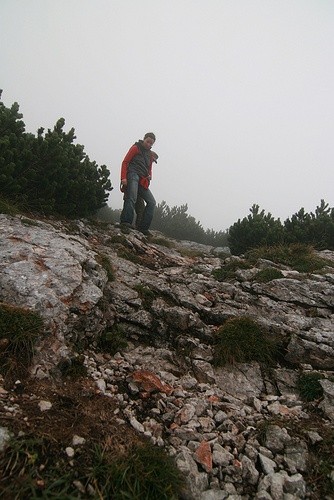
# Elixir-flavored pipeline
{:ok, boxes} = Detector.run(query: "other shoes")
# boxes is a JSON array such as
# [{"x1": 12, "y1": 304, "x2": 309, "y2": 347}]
[
  {"x1": 121, "y1": 223, "x2": 130, "y2": 233},
  {"x1": 140, "y1": 229, "x2": 154, "y2": 237}
]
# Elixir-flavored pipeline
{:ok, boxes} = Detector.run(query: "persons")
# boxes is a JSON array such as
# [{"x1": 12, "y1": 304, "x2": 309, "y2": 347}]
[{"x1": 120, "y1": 133, "x2": 159, "y2": 237}]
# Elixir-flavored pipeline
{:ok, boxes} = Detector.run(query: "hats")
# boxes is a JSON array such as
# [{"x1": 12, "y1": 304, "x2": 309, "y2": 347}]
[{"x1": 151, "y1": 151, "x2": 159, "y2": 164}]
